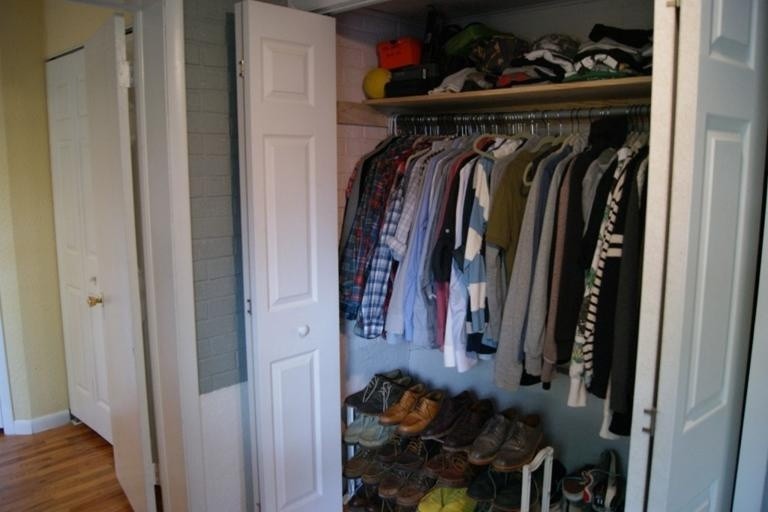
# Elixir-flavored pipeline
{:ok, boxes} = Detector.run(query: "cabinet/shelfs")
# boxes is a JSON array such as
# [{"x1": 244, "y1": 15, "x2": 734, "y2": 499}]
[
  {"x1": 350, "y1": 404, "x2": 553, "y2": 512},
  {"x1": 232, "y1": 0, "x2": 766, "y2": 512}
]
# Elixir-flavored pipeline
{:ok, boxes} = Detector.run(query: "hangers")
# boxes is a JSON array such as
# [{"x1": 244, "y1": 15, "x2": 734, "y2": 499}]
[{"x1": 382, "y1": 103, "x2": 649, "y2": 156}]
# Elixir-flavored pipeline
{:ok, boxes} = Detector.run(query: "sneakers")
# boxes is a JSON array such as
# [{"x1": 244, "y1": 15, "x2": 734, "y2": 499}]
[
  {"x1": 341, "y1": 415, "x2": 565, "y2": 512},
  {"x1": 561, "y1": 449, "x2": 622, "y2": 512},
  {"x1": 343, "y1": 369, "x2": 402, "y2": 405},
  {"x1": 359, "y1": 375, "x2": 415, "y2": 417},
  {"x1": 444, "y1": 412, "x2": 450, "y2": 413}
]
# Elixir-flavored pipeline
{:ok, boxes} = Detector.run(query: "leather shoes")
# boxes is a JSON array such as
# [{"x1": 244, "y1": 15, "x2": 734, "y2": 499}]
[
  {"x1": 379, "y1": 382, "x2": 427, "y2": 425},
  {"x1": 443, "y1": 398, "x2": 494, "y2": 452},
  {"x1": 495, "y1": 413, "x2": 545, "y2": 471},
  {"x1": 401, "y1": 388, "x2": 450, "y2": 433},
  {"x1": 470, "y1": 405, "x2": 521, "y2": 464},
  {"x1": 421, "y1": 390, "x2": 478, "y2": 440}
]
